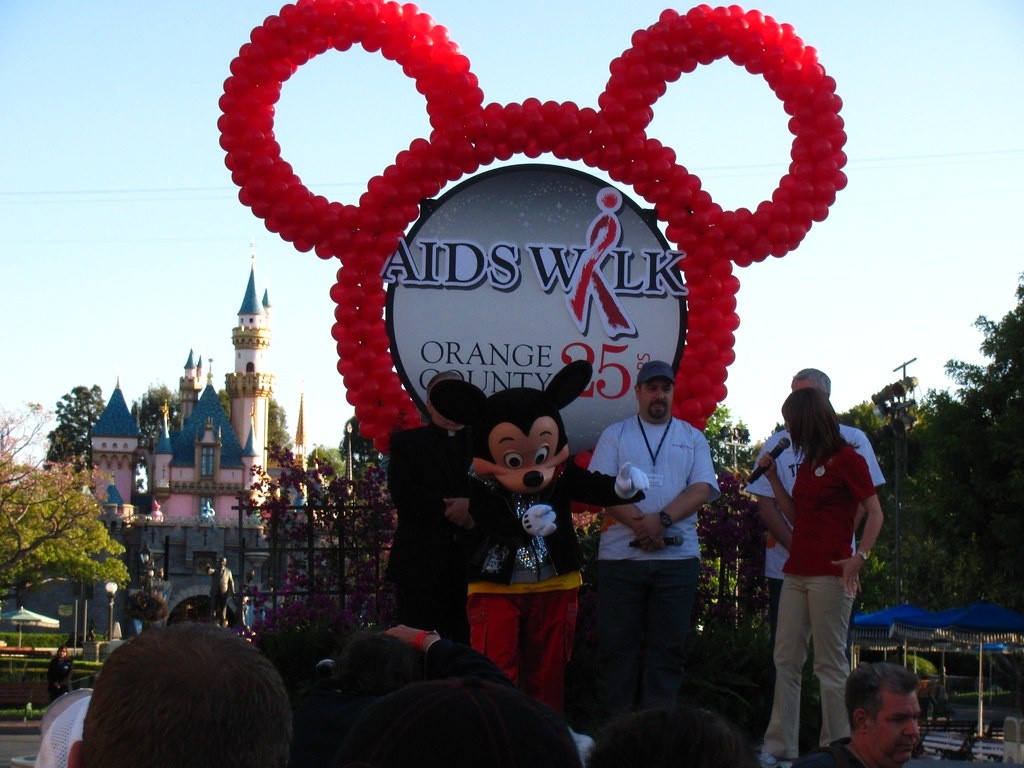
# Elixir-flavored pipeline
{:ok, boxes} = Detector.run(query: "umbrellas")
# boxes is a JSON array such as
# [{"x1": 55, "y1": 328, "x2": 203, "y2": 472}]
[
  {"x1": 0, "y1": 606, "x2": 60, "y2": 647},
  {"x1": 855, "y1": 600, "x2": 1024, "y2": 737}
]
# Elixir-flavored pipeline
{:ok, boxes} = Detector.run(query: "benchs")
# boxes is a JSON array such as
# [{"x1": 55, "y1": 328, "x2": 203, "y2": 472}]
[{"x1": 0, "y1": 681, "x2": 51, "y2": 719}]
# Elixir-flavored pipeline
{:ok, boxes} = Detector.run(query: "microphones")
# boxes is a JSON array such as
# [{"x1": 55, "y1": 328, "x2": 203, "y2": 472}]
[
  {"x1": 746, "y1": 437, "x2": 790, "y2": 484},
  {"x1": 629, "y1": 536, "x2": 683, "y2": 547}
]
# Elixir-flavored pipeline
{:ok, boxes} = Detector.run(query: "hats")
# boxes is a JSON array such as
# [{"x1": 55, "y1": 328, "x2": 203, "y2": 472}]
[{"x1": 637, "y1": 360, "x2": 675, "y2": 383}]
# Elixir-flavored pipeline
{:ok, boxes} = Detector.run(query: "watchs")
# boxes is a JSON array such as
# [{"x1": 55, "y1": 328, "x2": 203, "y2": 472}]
[
  {"x1": 858, "y1": 552, "x2": 866, "y2": 560},
  {"x1": 659, "y1": 511, "x2": 673, "y2": 527}
]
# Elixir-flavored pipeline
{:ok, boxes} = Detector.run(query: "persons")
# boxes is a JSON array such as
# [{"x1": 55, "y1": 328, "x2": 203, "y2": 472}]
[
  {"x1": 323, "y1": 625, "x2": 583, "y2": 768},
  {"x1": 588, "y1": 362, "x2": 722, "y2": 729},
  {"x1": 64, "y1": 621, "x2": 295, "y2": 768},
  {"x1": 791, "y1": 662, "x2": 921, "y2": 768},
  {"x1": 746, "y1": 369, "x2": 887, "y2": 768},
  {"x1": 46, "y1": 645, "x2": 73, "y2": 704},
  {"x1": 384, "y1": 370, "x2": 490, "y2": 649},
  {"x1": 584, "y1": 704, "x2": 754, "y2": 768},
  {"x1": 206, "y1": 557, "x2": 235, "y2": 626}
]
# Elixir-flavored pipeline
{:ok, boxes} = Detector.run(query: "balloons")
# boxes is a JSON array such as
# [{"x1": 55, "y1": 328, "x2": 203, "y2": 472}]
[{"x1": 217, "y1": 0, "x2": 848, "y2": 452}]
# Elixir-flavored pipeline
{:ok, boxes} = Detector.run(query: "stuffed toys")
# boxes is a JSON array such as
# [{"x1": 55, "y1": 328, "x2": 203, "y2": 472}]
[{"x1": 429, "y1": 359, "x2": 650, "y2": 712}]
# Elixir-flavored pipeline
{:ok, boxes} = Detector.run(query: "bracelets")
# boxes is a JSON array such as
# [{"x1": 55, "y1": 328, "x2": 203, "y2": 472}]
[{"x1": 413, "y1": 630, "x2": 434, "y2": 648}]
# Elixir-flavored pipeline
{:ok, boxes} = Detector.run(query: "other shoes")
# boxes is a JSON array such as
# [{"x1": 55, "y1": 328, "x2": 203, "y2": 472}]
[{"x1": 758, "y1": 753, "x2": 793, "y2": 768}]
